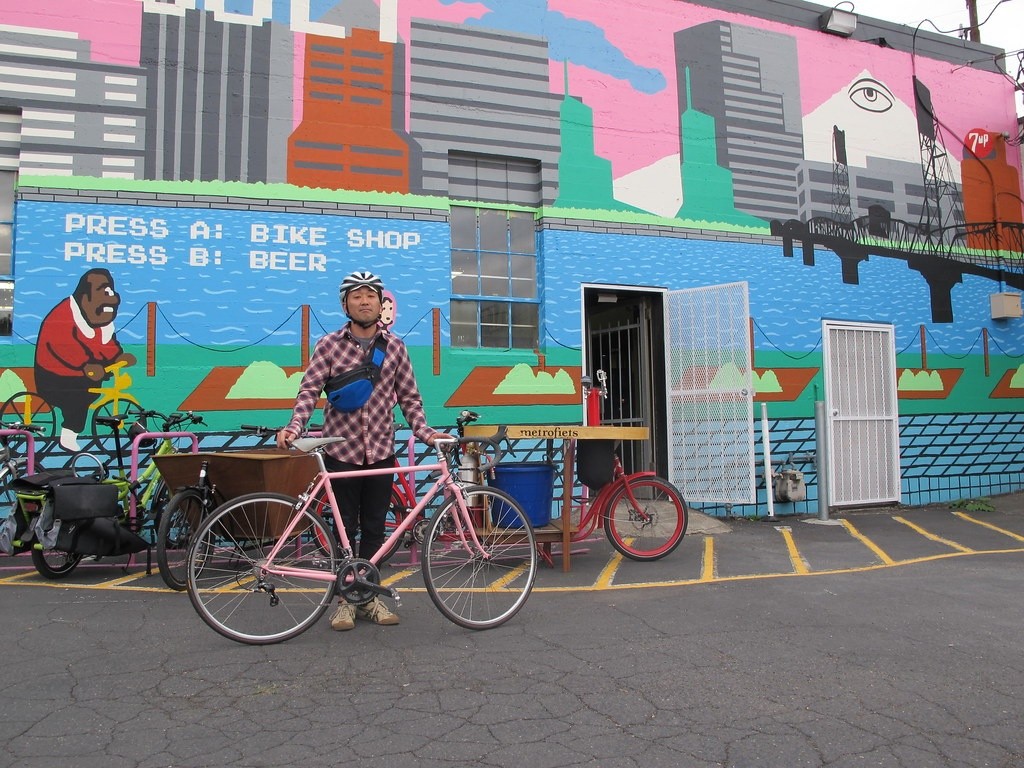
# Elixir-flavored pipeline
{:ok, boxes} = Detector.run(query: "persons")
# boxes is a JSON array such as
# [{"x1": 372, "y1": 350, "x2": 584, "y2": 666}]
[{"x1": 276, "y1": 271, "x2": 455, "y2": 631}]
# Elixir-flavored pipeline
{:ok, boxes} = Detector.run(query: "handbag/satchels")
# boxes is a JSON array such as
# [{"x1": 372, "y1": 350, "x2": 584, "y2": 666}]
[
  {"x1": 322, "y1": 332, "x2": 388, "y2": 413},
  {"x1": 34, "y1": 482, "x2": 152, "y2": 559},
  {"x1": 0, "y1": 501, "x2": 32, "y2": 556}
]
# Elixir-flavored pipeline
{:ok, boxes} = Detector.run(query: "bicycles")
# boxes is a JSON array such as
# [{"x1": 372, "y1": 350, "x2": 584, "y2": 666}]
[
  {"x1": 184, "y1": 425, "x2": 540, "y2": 646},
  {"x1": 1, "y1": 369, "x2": 690, "y2": 595}
]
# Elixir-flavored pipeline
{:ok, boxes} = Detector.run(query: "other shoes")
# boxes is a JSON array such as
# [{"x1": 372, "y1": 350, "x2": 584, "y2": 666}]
[
  {"x1": 355, "y1": 597, "x2": 399, "y2": 625},
  {"x1": 328, "y1": 599, "x2": 357, "y2": 632}
]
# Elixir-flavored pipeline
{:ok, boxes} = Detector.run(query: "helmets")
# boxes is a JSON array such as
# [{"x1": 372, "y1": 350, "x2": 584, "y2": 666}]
[
  {"x1": 128, "y1": 421, "x2": 159, "y2": 451},
  {"x1": 339, "y1": 270, "x2": 384, "y2": 301}
]
